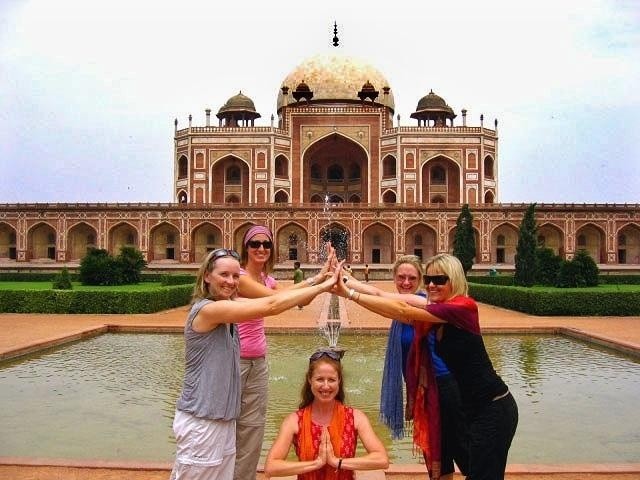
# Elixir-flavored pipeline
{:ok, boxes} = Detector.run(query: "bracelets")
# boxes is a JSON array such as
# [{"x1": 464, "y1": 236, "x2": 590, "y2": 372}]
[
  {"x1": 336, "y1": 457, "x2": 342, "y2": 471},
  {"x1": 348, "y1": 289, "x2": 355, "y2": 300},
  {"x1": 307, "y1": 278, "x2": 316, "y2": 286}
]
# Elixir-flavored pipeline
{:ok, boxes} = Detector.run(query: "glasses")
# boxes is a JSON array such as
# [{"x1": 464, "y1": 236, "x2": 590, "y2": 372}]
[
  {"x1": 208, "y1": 248, "x2": 240, "y2": 264},
  {"x1": 246, "y1": 240, "x2": 274, "y2": 249},
  {"x1": 423, "y1": 274, "x2": 450, "y2": 286},
  {"x1": 392, "y1": 273, "x2": 421, "y2": 283},
  {"x1": 309, "y1": 350, "x2": 343, "y2": 362}
]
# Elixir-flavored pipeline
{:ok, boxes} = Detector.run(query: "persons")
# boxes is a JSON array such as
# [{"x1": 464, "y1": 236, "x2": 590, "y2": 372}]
[
  {"x1": 329, "y1": 249, "x2": 455, "y2": 480},
  {"x1": 323, "y1": 253, "x2": 519, "y2": 480},
  {"x1": 293, "y1": 262, "x2": 303, "y2": 311},
  {"x1": 169, "y1": 247, "x2": 346, "y2": 480},
  {"x1": 347, "y1": 264, "x2": 352, "y2": 273},
  {"x1": 263, "y1": 348, "x2": 389, "y2": 480},
  {"x1": 230, "y1": 224, "x2": 335, "y2": 480},
  {"x1": 365, "y1": 264, "x2": 369, "y2": 282}
]
